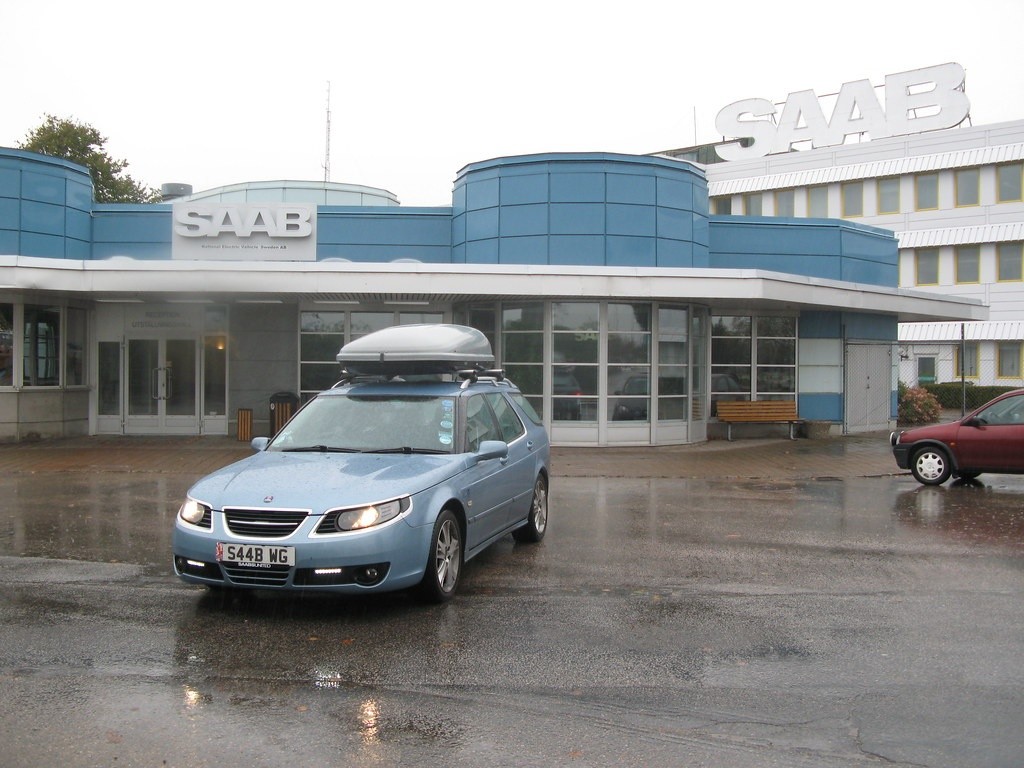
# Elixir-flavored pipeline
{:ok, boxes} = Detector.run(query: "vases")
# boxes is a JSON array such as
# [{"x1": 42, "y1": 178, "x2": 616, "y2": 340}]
[{"x1": 800, "y1": 419, "x2": 832, "y2": 440}]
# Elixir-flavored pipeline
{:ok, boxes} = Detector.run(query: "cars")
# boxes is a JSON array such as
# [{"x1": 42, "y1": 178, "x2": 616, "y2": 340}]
[{"x1": 889, "y1": 388, "x2": 1024, "y2": 485}]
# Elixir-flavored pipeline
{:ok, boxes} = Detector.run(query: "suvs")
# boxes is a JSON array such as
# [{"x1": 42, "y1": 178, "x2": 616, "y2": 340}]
[{"x1": 171, "y1": 324, "x2": 553, "y2": 601}]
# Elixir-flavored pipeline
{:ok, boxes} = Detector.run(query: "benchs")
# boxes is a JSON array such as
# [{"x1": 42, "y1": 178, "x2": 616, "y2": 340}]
[{"x1": 716, "y1": 401, "x2": 805, "y2": 442}]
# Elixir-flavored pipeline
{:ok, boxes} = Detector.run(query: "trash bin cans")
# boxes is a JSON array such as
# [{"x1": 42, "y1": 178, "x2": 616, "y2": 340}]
[{"x1": 268, "y1": 392, "x2": 301, "y2": 438}]
